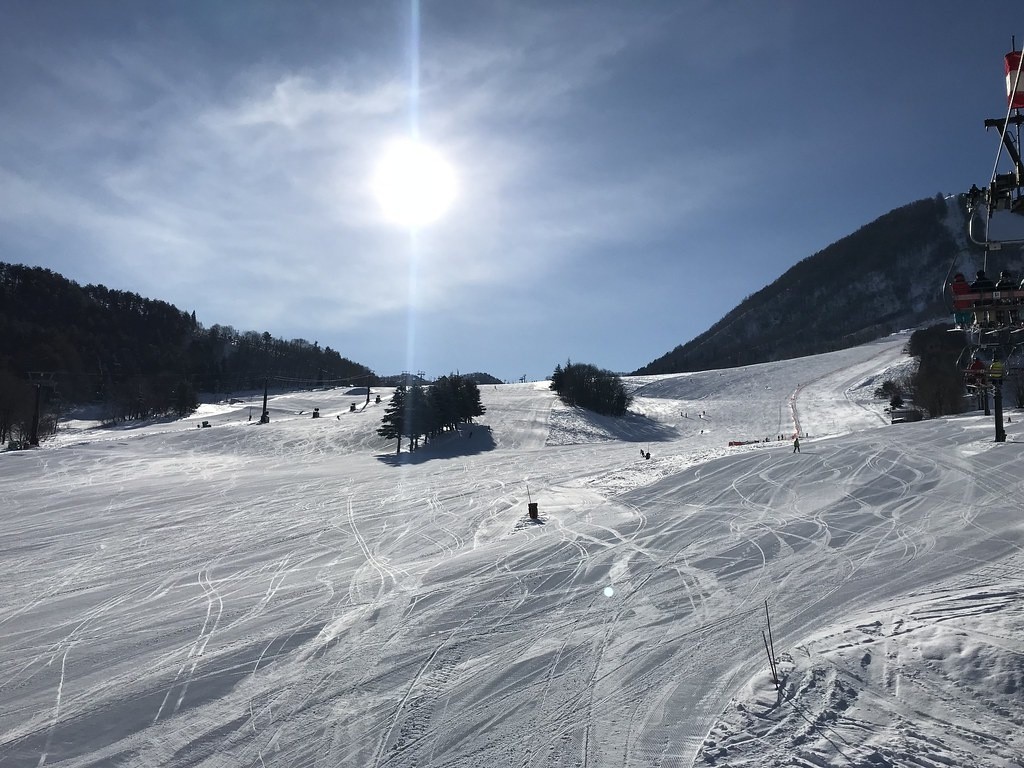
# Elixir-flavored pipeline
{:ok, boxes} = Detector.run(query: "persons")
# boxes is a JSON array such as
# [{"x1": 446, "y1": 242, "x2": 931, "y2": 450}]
[
  {"x1": 966, "y1": 184, "x2": 980, "y2": 207},
  {"x1": 951, "y1": 270, "x2": 1024, "y2": 329},
  {"x1": 966, "y1": 356, "x2": 1002, "y2": 395},
  {"x1": 640, "y1": 449, "x2": 644, "y2": 457},
  {"x1": 701, "y1": 430, "x2": 703, "y2": 434},
  {"x1": 793, "y1": 438, "x2": 800, "y2": 453},
  {"x1": 778, "y1": 434, "x2": 784, "y2": 441}
]
